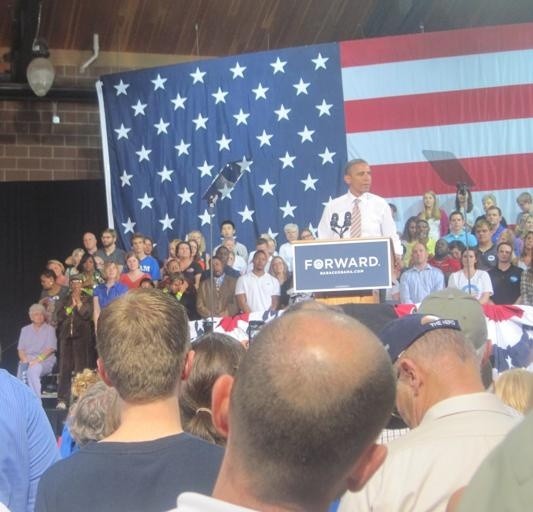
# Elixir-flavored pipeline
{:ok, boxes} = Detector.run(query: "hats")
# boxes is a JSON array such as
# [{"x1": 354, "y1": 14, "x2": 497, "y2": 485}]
[
  {"x1": 374, "y1": 313, "x2": 461, "y2": 365},
  {"x1": 69, "y1": 273, "x2": 83, "y2": 283}
]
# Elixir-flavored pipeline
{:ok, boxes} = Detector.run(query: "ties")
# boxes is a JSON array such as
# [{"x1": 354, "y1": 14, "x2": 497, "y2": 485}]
[
  {"x1": 215, "y1": 279, "x2": 221, "y2": 297},
  {"x1": 349, "y1": 199, "x2": 362, "y2": 240}
]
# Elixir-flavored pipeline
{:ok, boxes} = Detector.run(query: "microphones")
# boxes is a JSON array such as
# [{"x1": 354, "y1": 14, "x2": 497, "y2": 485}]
[
  {"x1": 330, "y1": 213, "x2": 338, "y2": 230},
  {"x1": 344, "y1": 211, "x2": 351, "y2": 230}
]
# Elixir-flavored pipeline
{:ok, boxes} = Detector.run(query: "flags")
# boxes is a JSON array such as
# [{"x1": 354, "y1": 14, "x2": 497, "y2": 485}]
[{"x1": 95, "y1": 22, "x2": 533, "y2": 267}]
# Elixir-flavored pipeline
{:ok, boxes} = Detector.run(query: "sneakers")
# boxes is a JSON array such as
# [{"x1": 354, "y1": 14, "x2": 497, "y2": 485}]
[{"x1": 56, "y1": 402, "x2": 67, "y2": 410}]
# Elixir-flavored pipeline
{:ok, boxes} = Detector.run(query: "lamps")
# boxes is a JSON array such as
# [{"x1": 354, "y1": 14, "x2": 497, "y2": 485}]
[{"x1": 26, "y1": 37, "x2": 55, "y2": 98}]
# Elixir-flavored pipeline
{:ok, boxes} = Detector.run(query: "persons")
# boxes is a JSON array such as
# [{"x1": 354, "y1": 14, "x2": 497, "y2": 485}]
[{"x1": 317, "y1": 158, "x2": 404, "y2": 271}]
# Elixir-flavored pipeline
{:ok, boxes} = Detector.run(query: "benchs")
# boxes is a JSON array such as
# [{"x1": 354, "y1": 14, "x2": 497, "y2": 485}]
[{"x1": 39, "y1": 371, "x2": 76, "y2": 400}]
